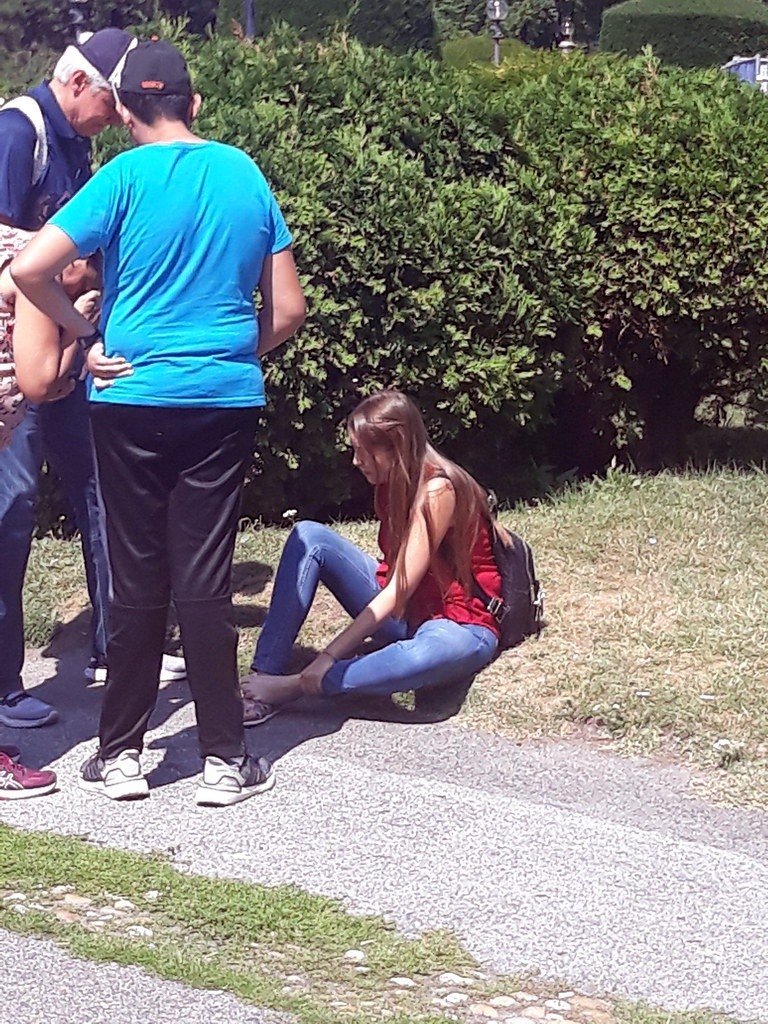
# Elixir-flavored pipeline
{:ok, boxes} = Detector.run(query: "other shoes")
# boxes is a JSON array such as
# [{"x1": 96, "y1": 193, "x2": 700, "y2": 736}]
[
  {"x1": 242, "y1": 696, "x2": 286, "y2": 725},
  {"x1": 84, "y1": 658, "x2": 108, "y2": 682},
  {"x1": 160, "y1": 653, "x2": 187, "y2": 682}
]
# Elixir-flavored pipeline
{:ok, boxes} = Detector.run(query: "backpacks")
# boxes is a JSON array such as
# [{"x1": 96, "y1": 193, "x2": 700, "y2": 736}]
[{"x1": 424, "y1": 471, "x2": 544, "y2": 651}]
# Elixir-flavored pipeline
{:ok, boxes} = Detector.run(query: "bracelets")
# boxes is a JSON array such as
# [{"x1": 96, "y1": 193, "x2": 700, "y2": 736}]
[
  {"x1": 76, "y1": 330, "x2": 102, "y2": 350},
  {"x1": 321, "y1": 652, "x2": 338, "y2": 664}
]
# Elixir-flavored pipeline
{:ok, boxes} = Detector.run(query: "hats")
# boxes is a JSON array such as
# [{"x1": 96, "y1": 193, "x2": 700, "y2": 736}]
[
  {"x1": 118, "y1": 35, "x2": 193, "y2": 95},
  {"x1": 73, "y1": 27, "x2": 142, "y2": 88}
]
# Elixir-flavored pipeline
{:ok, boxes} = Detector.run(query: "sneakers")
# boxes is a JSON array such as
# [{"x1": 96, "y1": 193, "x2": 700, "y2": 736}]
[
  {"x1": 195, "y1": 754, "x2": 277, "y2": 806},
  {"x1": 0, "y1": 690, "x2": 60, "y2": 729},
  {"x1": 77, "y1": 747, "x2": 150, "y2": 800},
  {"x1": 0, "y1": 752, "x2": 58, "y2": 801}
]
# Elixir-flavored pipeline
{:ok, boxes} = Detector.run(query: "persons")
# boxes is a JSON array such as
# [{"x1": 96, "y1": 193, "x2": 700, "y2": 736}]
[
  {"x1": 238, "y1": 391, "x2": 515, "y2": 726},
  {"x1": 0, "y1": 28, "x2": 189, "y2": 729},
  {"x1": 0, "y1": 221, "x2": 103, "y2": 801},
  {"x1": 8, "y1": 35, "x2": 311, "y2": 806}
]
